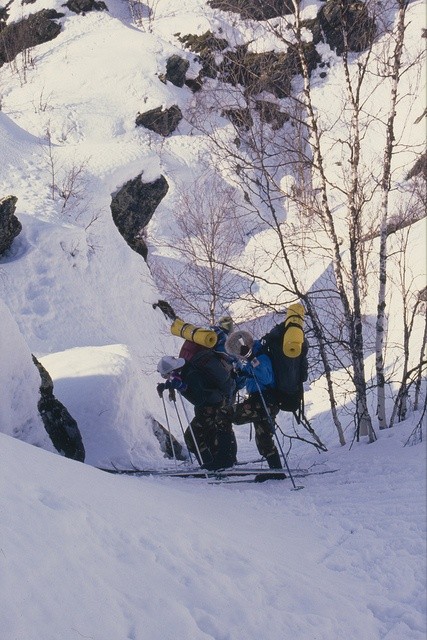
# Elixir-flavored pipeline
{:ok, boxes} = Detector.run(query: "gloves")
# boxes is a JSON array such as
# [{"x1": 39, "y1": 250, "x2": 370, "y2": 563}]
[
  {"x1": 168, "y1": 377, "x2": 187, "y2": 392},
  {"x1": 239, "y1": 361, "x2": 251, "y2": 374},
  {"x1": 157, "y1": 383, "x2": 169, "y2": 394},
  {"x1": 231, "y1": 367, "x2": 238, "y2": 378}
]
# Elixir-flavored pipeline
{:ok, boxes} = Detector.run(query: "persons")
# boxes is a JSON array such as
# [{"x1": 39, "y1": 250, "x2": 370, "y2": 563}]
[
  {"x1": 156, "y1": 356, "x2": 237, "y2": 471},
  {"x1": 198, "y1": 331, "x2": 285, "y2": 481}
]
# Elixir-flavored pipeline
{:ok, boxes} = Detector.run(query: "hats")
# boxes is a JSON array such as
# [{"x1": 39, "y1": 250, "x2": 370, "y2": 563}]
[
  {"x1": 226, "y1": 331, "x2": 252, "y2": 354},
  {"x1": 219, "y1": 316, "x2": 232, "y2": 327},
  {"x1": 158, "y1": 355, "x2": 185, "y2": 374}
]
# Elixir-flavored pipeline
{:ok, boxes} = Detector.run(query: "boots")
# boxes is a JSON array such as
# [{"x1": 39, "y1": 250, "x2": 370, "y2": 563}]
[
  {"x1": 194, "y1": 450, "x2": 216, "y2": 477},
  {"x1": 257, "y1": 450, "x2": 285, "y2": 483}
]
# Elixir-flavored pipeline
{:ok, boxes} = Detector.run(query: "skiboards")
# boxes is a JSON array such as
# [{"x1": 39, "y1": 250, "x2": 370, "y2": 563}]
[
  {"x1": 108, "y1": 460, "x2": 306, "y2": 476},
  {"x1": 204, "y1": 467, "x2": 340, "y2": 484}
]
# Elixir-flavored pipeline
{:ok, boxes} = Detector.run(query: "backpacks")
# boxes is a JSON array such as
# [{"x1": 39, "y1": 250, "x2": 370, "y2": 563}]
[
  {"x1": 249, "y1": 303, "x2": 308, "y2": 413},
  {"x1": 170, "y1": 321, "x2": 234, "y2": 392}
]
